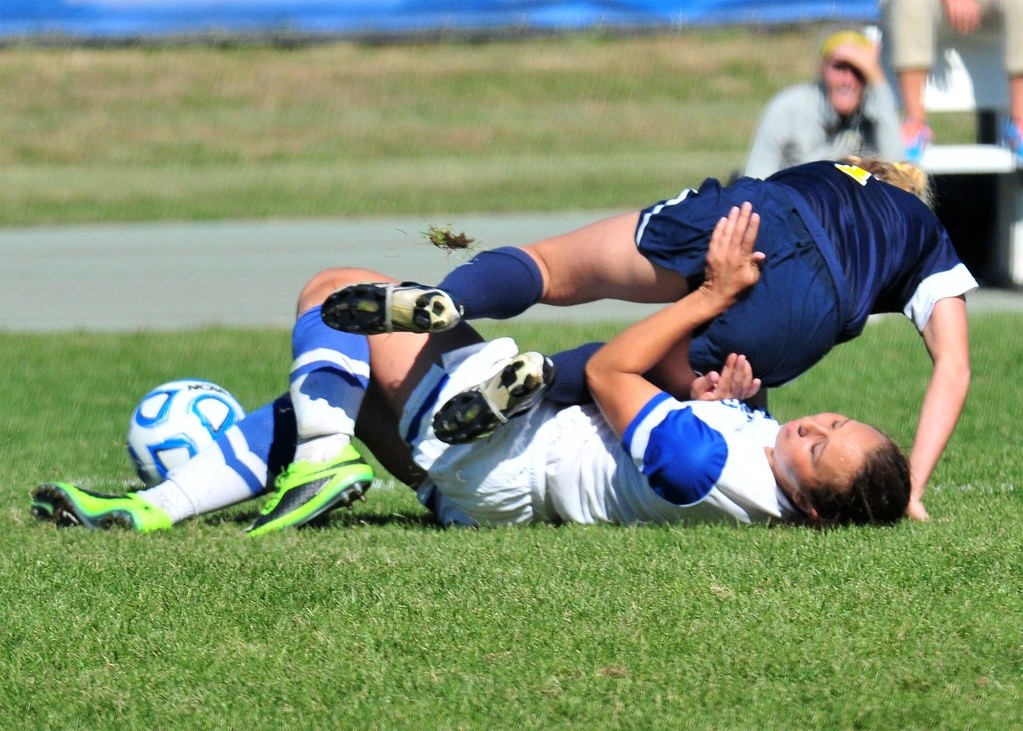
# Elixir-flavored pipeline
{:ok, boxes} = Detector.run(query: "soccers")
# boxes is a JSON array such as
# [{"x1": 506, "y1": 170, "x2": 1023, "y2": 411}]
[{"x1": 126, "y1": 378, "x2": 248, "y2": 489}]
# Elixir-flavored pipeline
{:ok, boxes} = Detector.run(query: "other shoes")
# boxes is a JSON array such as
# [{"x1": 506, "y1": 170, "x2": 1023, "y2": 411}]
[
  {"x1": 899, "y1": 123, "x2": 934, "y2": 163},
  {"x1": 997, "y1": 117, "x2": 1023, "y2": 171}
]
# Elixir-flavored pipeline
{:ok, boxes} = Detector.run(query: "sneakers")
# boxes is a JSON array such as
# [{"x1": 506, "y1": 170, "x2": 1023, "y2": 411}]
[
  {"x1": 320, "y1": 280, "x2": 465, "y2": 336},
  {"x1": 28, "y1": 480, "x2": 172, "y2": 533},
  {"x1": 241, "y1": 445, "x2": 373, "y2": 536},
  {"x1": 432, "y1": 351, "x2": 556, "y2": 445}
]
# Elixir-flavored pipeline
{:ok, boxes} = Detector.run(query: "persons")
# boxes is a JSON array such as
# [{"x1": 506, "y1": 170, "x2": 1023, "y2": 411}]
[
  {"x1": 30, "y1": 202, "x2": 913, "y2": 537},
  {"x1": 319, "y1": 156, "x2": 978, "y2": 522},
  {"x1": 880, "y1": 0, "x2": 1023, "y2": 293},
  {"x1": 743, "y1": 30, "x2": 902, "y2": 181}
]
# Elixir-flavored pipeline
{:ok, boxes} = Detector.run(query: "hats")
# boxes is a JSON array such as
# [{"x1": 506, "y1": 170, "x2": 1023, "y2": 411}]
[{"x1": 822, "y1": 31, "x2": 874, "y2": 63}]
